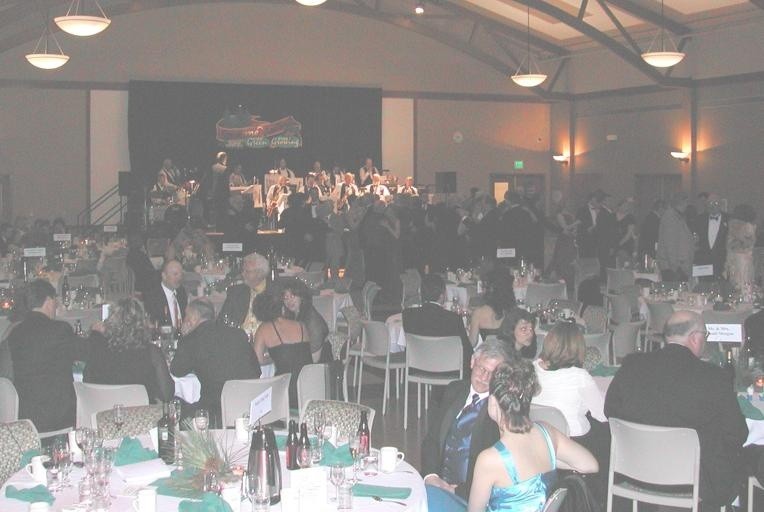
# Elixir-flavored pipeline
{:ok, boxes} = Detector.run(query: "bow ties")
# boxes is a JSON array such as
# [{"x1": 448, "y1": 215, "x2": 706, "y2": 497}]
[{"x1": 710, "y1": 216, "x2": 720, "y2": 220}]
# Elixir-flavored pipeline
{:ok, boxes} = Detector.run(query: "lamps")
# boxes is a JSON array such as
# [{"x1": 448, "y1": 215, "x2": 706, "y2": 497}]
[
  {"x1": 668, "y1": 150, "x2": 693, "y2": 165},
  {"x1": 509, "y1": 5, "x2": 548, "y2": 89},
  {"x1": 25, "y1": 1, "x2": 70, "y2": 71},
  {"x1": 550, "y1": 151, "x2": 571, "y2": 166},
  {"x1": 642, "y1": 0, "x2": 686, "y2": 71},
  {"x1": 53, "y1": 0, "x2": 111, "y2": 37}
]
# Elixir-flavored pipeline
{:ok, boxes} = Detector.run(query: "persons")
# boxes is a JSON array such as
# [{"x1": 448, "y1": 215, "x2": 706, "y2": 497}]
[
  {"x1": 1, "y1": 141, "x2": 764, "y2": 413},
  {"x1": 520, "y1": 318, "x2": 606, "y2": 441},
  {"x1": 172, "y1": 296, "x2": 261, "y2": 429},
  {"x1": 417, "y1": 337, "x2": 515, "y2": 500},
  {"x1": 466, "y1": 360, "x2": 603, "y2": 512},
  {"x1": 9, "y1": 276, "x2": 104, "y2": 438},
  {"x1": 603, "y1": 307, "x2": 752, "y2": 511}
]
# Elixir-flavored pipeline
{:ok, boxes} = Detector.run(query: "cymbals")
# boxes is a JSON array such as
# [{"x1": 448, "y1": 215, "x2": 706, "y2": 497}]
[{"x1": 152, "y1": 191, "x2": 173, "y2": 198}]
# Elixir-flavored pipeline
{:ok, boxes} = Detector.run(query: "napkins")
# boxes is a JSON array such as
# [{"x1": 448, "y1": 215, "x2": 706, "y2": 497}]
[
  {"x1": 4, "y1": 485, "x2": 54, "y2": 504},
  {"x1": 350, "y1": 482, "x2": 413, "y2": 499},
  {"x1": 104, "y1": 436, "x2": 160, "y2": 469},
  {"x1": 317, "y1": 438, "x2": 359, "y2": 467},
  {"x1": 273, "y1": 431, "x2": 316, "y2": 453}
]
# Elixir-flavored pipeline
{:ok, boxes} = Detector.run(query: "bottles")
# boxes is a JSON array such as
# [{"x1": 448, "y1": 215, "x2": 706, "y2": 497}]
[
  {"x1": 74, "y1": 319, "x2": 84, "y2": 338},
  {"x1": 746, "y1": 386, "x2": 754, "y2": 402},
  {"x1": 654, "y1": 280, "x2": 744, "y2": 310},
  {"x1": 759, "y1": 389, "x2": 764, "y2": 401},
  {"x1": 335, "y1": 487, "x2": 353, "y2": 512},
  {"x1": 535, "y1": 300, "x2": 559, "y2": 328},
  {"x1": 61, "y1": 275, "x2": 71, "y2": 307},
  {"x1": 515, "y1": 299, "x2": 526, "y2": 310},
  {"x1": 742, "y1": 332, "x2": 755, "y2": 368},
  {"x1": 449, "y1": 296, "x2": 468, "y2": 316},
  {"x1": 157, "y1": 398, "x2": 178, "y2": 465},
  {"x1": 285, "y1": 411, "x2": 309, "y2": 470},
  {"x1": 518, "y1": 256, "x2": 525, "y2": 277},
  {"x1": 221, "y1": 313, "x2": 253, "y2": 345},
  {"x1": 0, "y1": 286, "x2": 15, "y2": 315},
  {"x1": 723, "y1": 349, "x2": 737, "y2": 396},
  {"x1": 358, "y1": 411, "x2": 370, "y2": 456}
]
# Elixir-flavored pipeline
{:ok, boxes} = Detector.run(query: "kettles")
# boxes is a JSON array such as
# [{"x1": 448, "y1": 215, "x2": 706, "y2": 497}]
[{"x1": 245, "y1": 424, "x2": 281, "y2": 505}]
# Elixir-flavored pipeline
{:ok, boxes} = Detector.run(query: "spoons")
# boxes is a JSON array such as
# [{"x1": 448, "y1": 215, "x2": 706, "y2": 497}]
[{"x1": 370, "y1": 495, "x2": 408, "y2": 507}]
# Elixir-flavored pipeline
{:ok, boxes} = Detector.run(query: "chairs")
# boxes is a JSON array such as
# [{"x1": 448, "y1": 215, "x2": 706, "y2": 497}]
[
  {"x1": 388, "y1": 242, "x2": 764, "y2": 396},
  {"x1": 403, "y1": 331, "x2": 464, "y2": 429},
  {"x1": 220, "y1": 373, "x2": 292, "y2": 432},
  {"x1": 525, "y1": 404, "x2": 570, "y2": 436},
  {"x1": 359, "y1": 315, "x2": 406, "y2": 413},
  {"x1": 91, "y1": 402, "x2": 166, "y2": 434},
  {"x1": 72, "y1": 379, "x2": 150, "y2": 430},
  {"x1": 1, "y1": 418, "x2": 43, "y2": 484},
  {"x1": 297, "y1": 397, "x2": 376, "y2": 447},
  {"x1": 337, "y1": 278, "x2": 384, "y2": 342},
  {"x1": 296, "y1": 361, "x2": 333, "y2": 414},
  {"x1": 0, "y1": 240, "x2": 353, "y2": 403},
  {"x1": 605, "y1": 415, "x2": 702, "y2": 512}
]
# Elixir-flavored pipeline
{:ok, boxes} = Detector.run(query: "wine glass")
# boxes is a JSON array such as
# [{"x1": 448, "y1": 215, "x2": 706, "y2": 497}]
[
  {"x1": 349, "y1": 428, "x2": 359, "y2": 483},
  {"x1": 52, "y1": 402, "x2": 127, "y2": 507},
  {"x1": 149, "y1": 316, "x2": 180, "y2": 361},
  {"x1": 62, "y1": 295, "x2": 70, "y2": 313},
  {"x1": 195, "y1": 408, "x2": 211, "y2": 437},
  {"x1": 197, "y1": 251, "x2": 295, "y2": 275},
  {"x1": 327, "y1": 462, "x2": 346, "y2": 503},
  {"x1": 294, "y1": 275, "x2": 318, "y2": 291},
  {"x1": 3, "y1": 240, "x2": 86, "y2": 277}
]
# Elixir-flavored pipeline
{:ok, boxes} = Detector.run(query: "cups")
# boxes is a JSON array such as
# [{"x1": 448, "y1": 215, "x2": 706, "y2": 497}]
[
  {"x1": 131, "y1": 488, "x2": 157, "y2": 512},
  {"x1": 561, "y1": 308, "x2": 575, "y2": 319},
  {"x1": 455, "y1": 266, "x2": 465, "y2": 283},
  {"x1": 234, "y1": 418, "x2": 251, "y2": 440},
  {"x1": 379, "y1": 445, "x2": 405, "y2": 473},
  {"x1": 25, "y1": 454, "x2": 52, "y2": 488},
  {"x1": 312, "y1": 409, "x2": 329, "y2": 465},
  {"x1": 294, "y1": 445, "x2": 314, "y2": 469},
  {"x1": 359, "y1": 453, "x2": 379, "y2": 482},
  {"x1": 643, "y1": 286, "x2": 651, "y2": 297},
  {"x1": 241, "y1": 472, "x2": 271, "y2": 510}
]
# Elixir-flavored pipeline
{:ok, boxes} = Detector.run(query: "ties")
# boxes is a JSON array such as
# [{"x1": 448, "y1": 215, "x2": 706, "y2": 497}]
[
  {"x1": 248, "y1": 289, "x2": 256, "y2": 336},
  {"x1": 171, "y1": 293, "x2": 178, "y2": 329},
  {"x1": 471, "y1": 394, "x2": 480, "y2": 405}
]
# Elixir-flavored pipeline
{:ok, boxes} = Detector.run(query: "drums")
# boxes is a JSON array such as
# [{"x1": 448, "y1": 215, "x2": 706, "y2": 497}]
[{"x1": 154, "y1": 203, "x2": 187, "y2": 227}]
